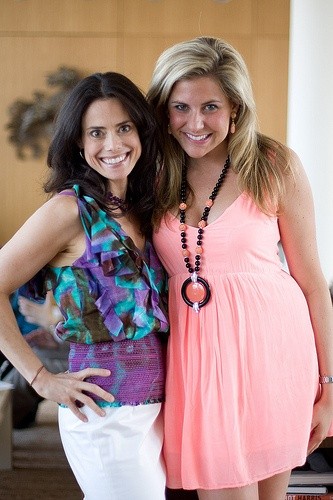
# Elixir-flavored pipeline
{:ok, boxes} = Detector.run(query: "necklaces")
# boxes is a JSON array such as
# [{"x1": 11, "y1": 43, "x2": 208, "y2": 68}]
[{"x1": 179, "y1": 155, "x2": 232, "y2": 308}]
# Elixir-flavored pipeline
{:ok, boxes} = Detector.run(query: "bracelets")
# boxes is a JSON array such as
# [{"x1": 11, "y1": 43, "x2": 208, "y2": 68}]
[
  {"x1": 30, "y1": 365, "x2": 44, "y2": 386},
  {"x1": 319, "y1": 376, "x2": 333, "y2": 383}
]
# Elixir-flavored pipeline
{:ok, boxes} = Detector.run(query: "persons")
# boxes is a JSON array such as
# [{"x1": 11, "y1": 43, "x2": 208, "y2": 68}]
[
  {"x1": 18, "y1": 36, "x2": 333, "y2": 500},
  {"x1": 0, "y1": 72, "x2": 167, "y2": 500}
]
